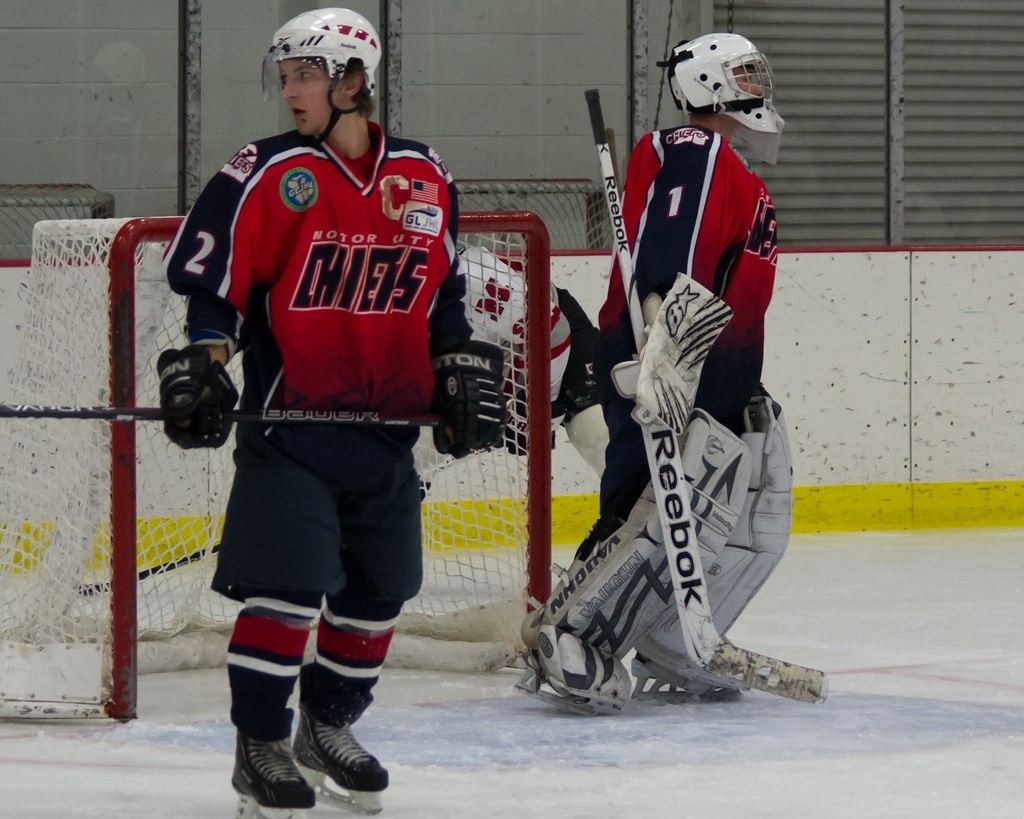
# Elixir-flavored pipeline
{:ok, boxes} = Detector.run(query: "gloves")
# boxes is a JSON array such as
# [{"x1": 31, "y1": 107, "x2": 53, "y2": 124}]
[
  {"x1": 156, "y1": 344, "x2": 238, "y2": 450},
  {"x1": 430, "y1": 335, "x2": 510, "y2": 457}
]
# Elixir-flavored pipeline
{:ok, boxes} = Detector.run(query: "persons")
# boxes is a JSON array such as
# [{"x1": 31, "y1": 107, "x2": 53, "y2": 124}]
[
  {"x1": 409, "y1": 230, "x2": 617, "y2": 492},
  {"x1": 517, "y1": 29, "x2": 792, "y2": 703},
  {"x1": 158, "y1": 14, "x2": 509, "y2": 819}
]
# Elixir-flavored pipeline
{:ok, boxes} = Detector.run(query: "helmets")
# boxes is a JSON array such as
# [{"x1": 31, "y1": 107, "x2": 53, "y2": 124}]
[
  {"x1": 657, "y1": 31, "x2": 786, "y2": 134},
  {"x1": 259, "y1": 7, "x2": 382, "y2": 102}
]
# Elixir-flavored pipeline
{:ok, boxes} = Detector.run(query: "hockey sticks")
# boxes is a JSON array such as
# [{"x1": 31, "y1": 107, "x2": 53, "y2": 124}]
[
  {"x1": 73, "y1": 449, "x2": 478, "y2": 595},
  {"x1": 584, "y1": 87, "x2": 824, "y2": 704},
  {"x1": 0, "y1": 404, "x2": 440, "y2": 425}
]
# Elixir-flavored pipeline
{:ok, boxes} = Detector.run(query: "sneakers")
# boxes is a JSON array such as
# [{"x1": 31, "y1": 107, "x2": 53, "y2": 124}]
[
  {"x1": 231, "y1": 721, "x2": 318, "y2": 809},
  {"x1": 294, "y1": 702, "x2": 389, "y2": 790}
]
